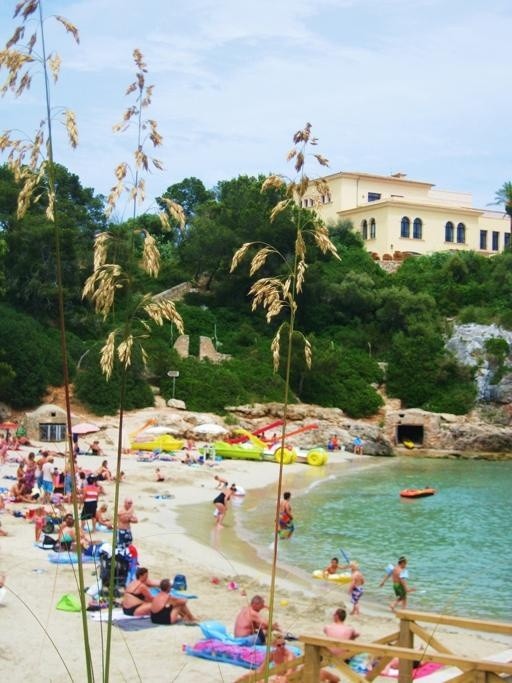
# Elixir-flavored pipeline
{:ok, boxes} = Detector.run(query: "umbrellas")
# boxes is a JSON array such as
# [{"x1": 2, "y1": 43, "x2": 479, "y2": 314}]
[
  {"x1": 0, "y1": 420, "x2": 19, "y2": 442},
  {"x1": 146, "y1": 427, "x2": 180, "y2": 459},
  {"x1": 71, "y1": 423, "x2": 102, "y2": 446},
  {"x1": 189, "y1": 424, "x2": 230, "y2": 443}
]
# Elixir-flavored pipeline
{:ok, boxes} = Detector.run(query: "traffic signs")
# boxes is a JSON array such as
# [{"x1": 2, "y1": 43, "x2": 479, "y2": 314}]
[{"x1": 166, "y1": 369, "x2": 180, "y2": 377}]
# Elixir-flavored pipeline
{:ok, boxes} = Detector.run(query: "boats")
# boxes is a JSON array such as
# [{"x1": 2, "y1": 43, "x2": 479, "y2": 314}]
[
  {"x1": 397, "y1": 487, "x2": 435, "y2": 497},
  {"x1": 313, "y1": 568, "x2": 353, "y2": 582},
  {"x1": 130, "y1": 419, "x2": 329, "y2": 465},
  {"x1": 399, "y1": 435, "x2": 415, "y2": 448}
]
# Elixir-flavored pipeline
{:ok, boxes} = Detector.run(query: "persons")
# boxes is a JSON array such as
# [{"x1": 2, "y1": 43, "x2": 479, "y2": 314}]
[
  {"x1": 347, "y1": 559, "x2": 364, "y2": 616},
  {"x1": 259, "y1": 431, "x2": 267, "y2": 441},
  {"x1": 233, "y1": 595, "x2": 281, "y2": 639},
  {"x1": 1, "y1": 434, "x2": 207, "y2": 624},
  {"x1": 285, "y1": 654, "x2": 341, "y2": 683},
  {"x1": 324, "y1": 557, "x2": 351, "y2": 575},
  {"x1": 331, "y1": 434, "x2": 341, "y2": 450},
  {"x1": 383, "y1": 643, "x2": 426, "y2": 673},
  {"x1": 213, "y1": 483, "x2": 237, "y2": 528},
  {"x1": 228, "y1": 483, "x2": 236, "y2": 500},
  {"x1": 352, "y1": 434, "x2": 365, "y2": 454},
  {"x1": 214, "y1": 474, "x2": 228, "y2": 490},
  {"x1": 273, "y1": 490, "x2": 296, "y2": 539},
  {"x1": 327, "y1": 437, "x2": 334, "y2": 451},
  {"x1": 322, "y1": 607, "x2": 360, "y2": 656},
  {"x1": 271, "y1": 432, "x2": 279, "y2": 443},
  {"x1": 378, "y1": 555, "x2": 417, "y2": 613},
  {"x1": 254, "y1": 632, "x2": 296, "y2": 683}
]
